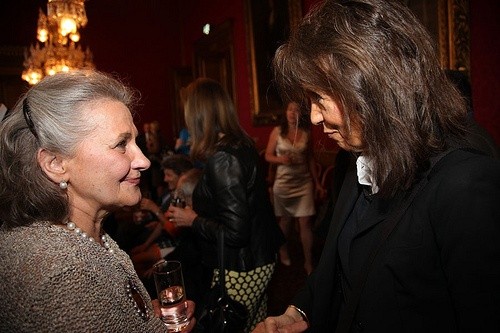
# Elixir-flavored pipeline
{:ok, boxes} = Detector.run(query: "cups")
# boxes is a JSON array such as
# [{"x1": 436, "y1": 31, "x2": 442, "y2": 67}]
[
  {"x1": 171, "y1": 190, "x2": 187, "y2": 209},
  {"x1": 153, "y1": 261, "x2": 191, "y2": 333}
]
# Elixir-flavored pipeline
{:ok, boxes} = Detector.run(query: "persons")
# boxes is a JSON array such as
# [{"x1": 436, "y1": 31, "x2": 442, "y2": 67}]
[
  {"x1": 0, "y1": 68, "x2": 323, "y2": 333},
  {"x1": 265, "y1": 0, "x2": 500, "y2": 333}
]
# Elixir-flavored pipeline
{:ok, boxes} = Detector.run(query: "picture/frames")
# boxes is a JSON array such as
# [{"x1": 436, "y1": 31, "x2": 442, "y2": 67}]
[
  {"x1": 404, "y1": 0, "x2": 473, "y2": 85},
  {"x1": 195, "y1": 40, "x2": 235, "y2": 112},
  {"x1": 244, "y1": 0, "x2": 314, "y2": 127}
]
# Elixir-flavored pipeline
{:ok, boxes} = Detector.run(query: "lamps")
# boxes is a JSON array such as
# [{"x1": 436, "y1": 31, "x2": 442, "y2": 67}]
[{"x1": 21, "y1": 0, "x2": 97, "y2": 87}]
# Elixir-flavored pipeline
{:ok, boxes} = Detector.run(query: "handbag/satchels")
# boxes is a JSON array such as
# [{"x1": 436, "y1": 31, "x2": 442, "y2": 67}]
[{"x1": 197, "y1": 286, "x2": 249, "y2": 333}]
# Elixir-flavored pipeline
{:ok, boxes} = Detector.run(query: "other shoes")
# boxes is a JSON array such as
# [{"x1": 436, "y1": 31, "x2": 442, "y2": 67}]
[{"x1": 280, "y1": 250, "x2": 290, "y2": 265}]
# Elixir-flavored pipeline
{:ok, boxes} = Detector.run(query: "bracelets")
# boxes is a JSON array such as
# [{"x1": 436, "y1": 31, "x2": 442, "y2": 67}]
[
  {"x1": 155, "y1": 209, "x2": 162, "y2": 216},
  {"x1": 288, "y1": 304, "x2": 310, "y2": 326}
]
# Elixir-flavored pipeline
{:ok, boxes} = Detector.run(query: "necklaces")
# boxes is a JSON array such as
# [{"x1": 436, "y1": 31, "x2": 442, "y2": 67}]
[{"x1": 64, "y1": 219, "x2": 151, "y2": 319}]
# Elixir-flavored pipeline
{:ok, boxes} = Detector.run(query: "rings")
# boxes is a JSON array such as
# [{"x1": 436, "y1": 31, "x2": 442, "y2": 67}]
[
  {"x1": 169, "y1": 218, "x2": 174, "y2": 222},
  {"x1": 168, "y1": 211, "x2": 174, "y2": 219}
]
